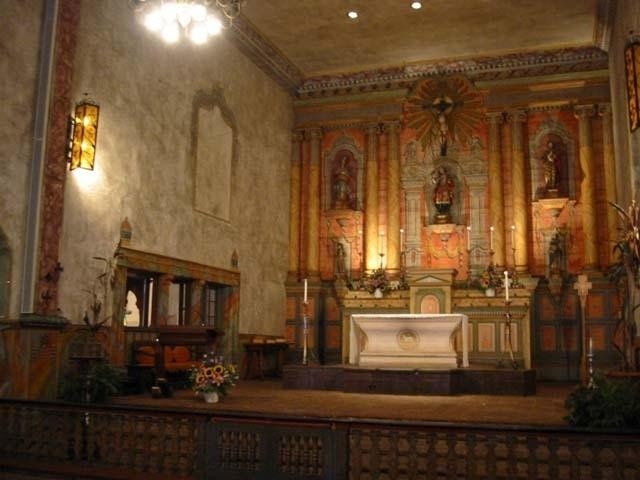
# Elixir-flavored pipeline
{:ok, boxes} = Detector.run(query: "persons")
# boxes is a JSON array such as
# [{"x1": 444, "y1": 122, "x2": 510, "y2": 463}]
[
  {"x1": 429, "y1": 97, "x2": 454, "y2": 145},
  {"x1": 332, "y1": 237, "x2": 347, "y2": 272},
  {"x1": 543, "y1": 215, "x2": 568, "y2": 269},
  {"x1": 330, "y1": 155, "x2": 355, "y2": 201},
  {"x1": 429, "y1": 166, "x2": 456, "y2": 214},
  {"x1": 540, "y1": 141, "x2": 560, "y2": 189}
]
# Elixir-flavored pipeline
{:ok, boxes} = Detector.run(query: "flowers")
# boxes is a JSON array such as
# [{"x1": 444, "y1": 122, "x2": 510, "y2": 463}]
[
  {"x1": 185, "y1": 350, "x2": 241, "y2": 402},
  {"x1": 360, "y1": 265, "x2": 401, "y2": 296},
  {"x1": 475, "y1": 262, "x2": 503, "y2": 295}
]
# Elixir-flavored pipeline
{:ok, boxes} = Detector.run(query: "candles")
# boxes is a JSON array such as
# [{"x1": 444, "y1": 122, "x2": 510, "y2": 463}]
[
  {"x1": 512, "y1": 230, "x2": 514, "y2": 248},
  {"x1": 303, "y1": 278, "x2": 308, "y2": 302},
  {"x1": 490, "y1": 231, "x2": 493, "y2": 249},
  {"x1": 467, "y1": 230, "x2": 470, "y2": 250},
  {"x1": 400, "y1": 233, "x2": 403, "y2": 252},
  {"x1": 504, "y1": 274, "x2": 509, "y2": 300}
]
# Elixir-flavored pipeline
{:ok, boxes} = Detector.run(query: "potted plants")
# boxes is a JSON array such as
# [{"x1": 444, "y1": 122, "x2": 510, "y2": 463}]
[
  {"x1": 54, "y1": 239, "x2": 128, "y2": 410},
  {"x1": 562, "y1": 192, "x2": 640, "y2": 431}
]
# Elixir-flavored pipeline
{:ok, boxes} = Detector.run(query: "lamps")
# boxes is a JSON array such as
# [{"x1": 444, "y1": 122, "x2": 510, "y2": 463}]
[{"x1": 65, "y1": 90, "x2": 100, "y2": 169}]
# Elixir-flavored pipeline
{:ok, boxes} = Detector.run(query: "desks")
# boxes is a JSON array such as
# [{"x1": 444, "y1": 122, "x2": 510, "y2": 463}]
[{"x1": 241, "y1": 341, "x2": 293, "y2": 381}]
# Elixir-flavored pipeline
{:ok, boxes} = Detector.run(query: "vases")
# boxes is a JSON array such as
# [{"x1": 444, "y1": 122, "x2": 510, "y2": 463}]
[
  {"x1": 202, "y1": 393, "x2": 218, "y2": 402},
  {"x1": 484, "y1": 288, "x2": 495, "y2": 298},
  {"x1": 374, "y1": 286, "x2": 383, "y2": 297}
]
